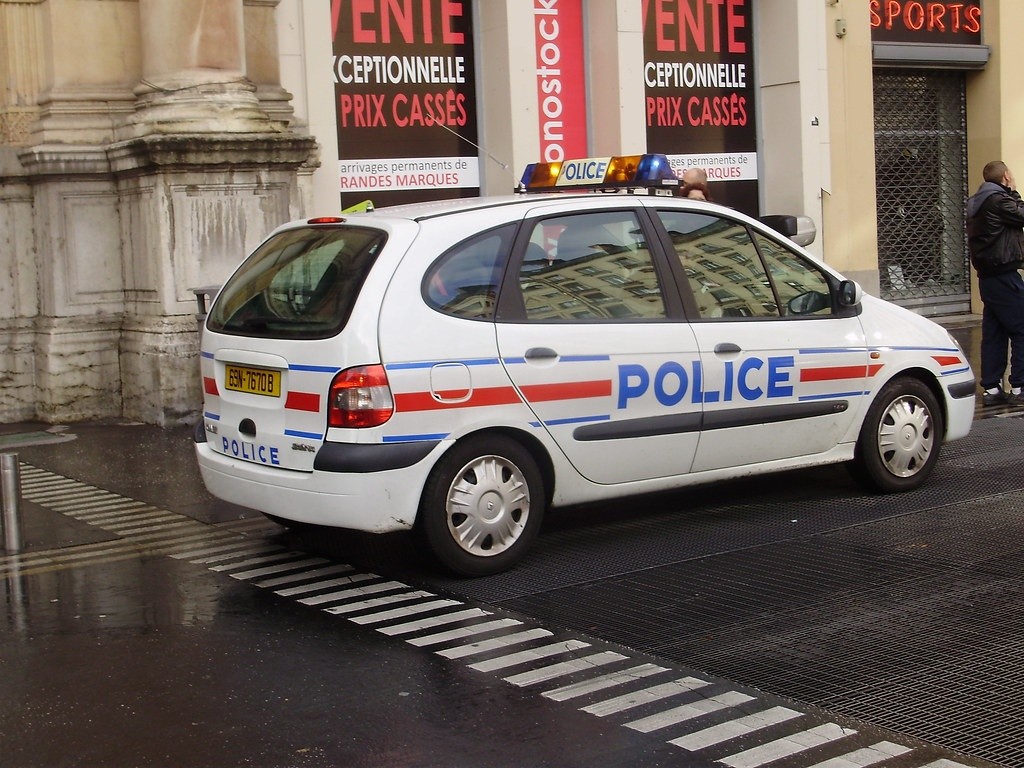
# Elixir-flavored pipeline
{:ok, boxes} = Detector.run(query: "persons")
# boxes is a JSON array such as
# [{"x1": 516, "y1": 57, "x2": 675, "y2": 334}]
[
  {"x1": 967, "y1": 160, "x2": 1024, "y2": 407},
  {"x1": 669, "y1": 183, "x2": 717, "y2": 236}
]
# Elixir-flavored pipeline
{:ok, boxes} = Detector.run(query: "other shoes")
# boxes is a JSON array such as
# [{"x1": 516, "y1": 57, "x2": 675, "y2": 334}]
[
  {"x1": 983, "y1": 385, "x2": 1010, "y2": 405},
  {"x1": 1007, "y1": 389, "x2": 1024, "y2": 407}
]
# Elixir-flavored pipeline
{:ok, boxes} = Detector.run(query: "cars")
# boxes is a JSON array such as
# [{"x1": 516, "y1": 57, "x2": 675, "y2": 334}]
[{"x1": 192, "y1": 154, "x2": 977, "y2": 575}]
